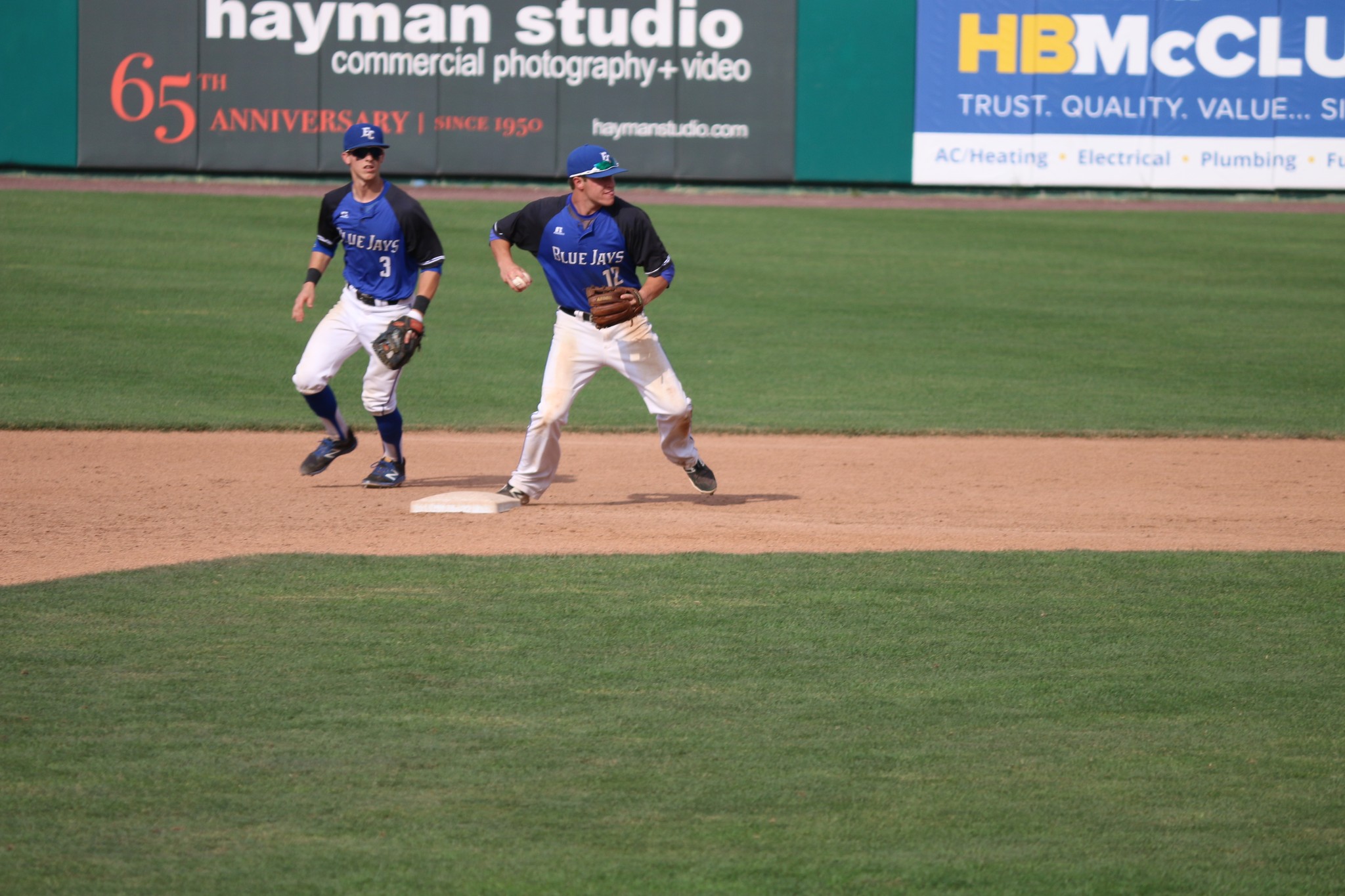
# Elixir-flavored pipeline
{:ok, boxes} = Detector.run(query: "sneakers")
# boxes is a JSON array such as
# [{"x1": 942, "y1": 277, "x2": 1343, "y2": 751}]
[
  {"x1": 362, "y1": 457, "x2": 406, "y2": 489},
  {"x1": 496, "y1": 483, "x2": 529, "y2": 504},
  {"x1": 299, "y1": 428, "x2": 358, "y2": 476},
  {"x1": 683, "y1": 457, "x2": 717, "y2": 493}
]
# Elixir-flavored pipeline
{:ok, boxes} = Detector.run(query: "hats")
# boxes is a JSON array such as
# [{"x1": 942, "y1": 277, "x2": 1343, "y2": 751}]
[
  {"x1": 342, "y1": 123, "x2": 391, "y2": 152},
  {"x1": 566, "y1": 143, "x2": 629, "y2": 179}
]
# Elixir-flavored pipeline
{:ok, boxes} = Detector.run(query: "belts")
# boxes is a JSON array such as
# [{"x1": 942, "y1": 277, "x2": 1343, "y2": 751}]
[
  {"x1": 347, "y1": 284, "x2": 399, "y2": 306},
  {"x1": 559, "y1": 305, "x2": 589, "y2": 321}
]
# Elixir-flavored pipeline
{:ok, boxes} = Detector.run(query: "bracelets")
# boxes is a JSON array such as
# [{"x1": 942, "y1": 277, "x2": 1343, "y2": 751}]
[
  {"x1": 411, "y1": 295, "x2": 431, "y2": 314},
  {"x1": 304, "y1": 268, "x2": 322, "y2": 286}
]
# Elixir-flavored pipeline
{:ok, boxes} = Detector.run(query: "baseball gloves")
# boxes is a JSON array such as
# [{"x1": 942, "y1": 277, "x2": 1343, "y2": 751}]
[
  {"x1": 584, "y1": 286, "x2": 642, "y2": 330},
  {"x1": 373, "y1": 316, "x2": 425, "y2": 370}
]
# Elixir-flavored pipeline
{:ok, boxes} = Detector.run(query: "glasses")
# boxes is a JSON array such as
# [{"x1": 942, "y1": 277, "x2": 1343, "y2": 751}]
[
  {"x1": 347, "y1": 146, "x2": 383, "y2": 158},
  {"x1": 569, "y1": 155, "x2": 620, "y2": 177}
]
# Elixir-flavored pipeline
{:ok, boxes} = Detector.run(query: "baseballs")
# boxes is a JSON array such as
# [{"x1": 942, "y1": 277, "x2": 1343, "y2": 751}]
[{"x1": 514, "y1": 273, "x2": 530, "y2": 289}]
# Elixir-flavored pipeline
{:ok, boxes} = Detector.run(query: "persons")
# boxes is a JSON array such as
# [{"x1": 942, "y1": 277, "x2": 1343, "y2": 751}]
[
  {"x1": 290, "y1": 123, "x2": 444, "y2": 491},
  {"x1": 486, "y1": 145, "x2": 717, "y2": 504}
]
